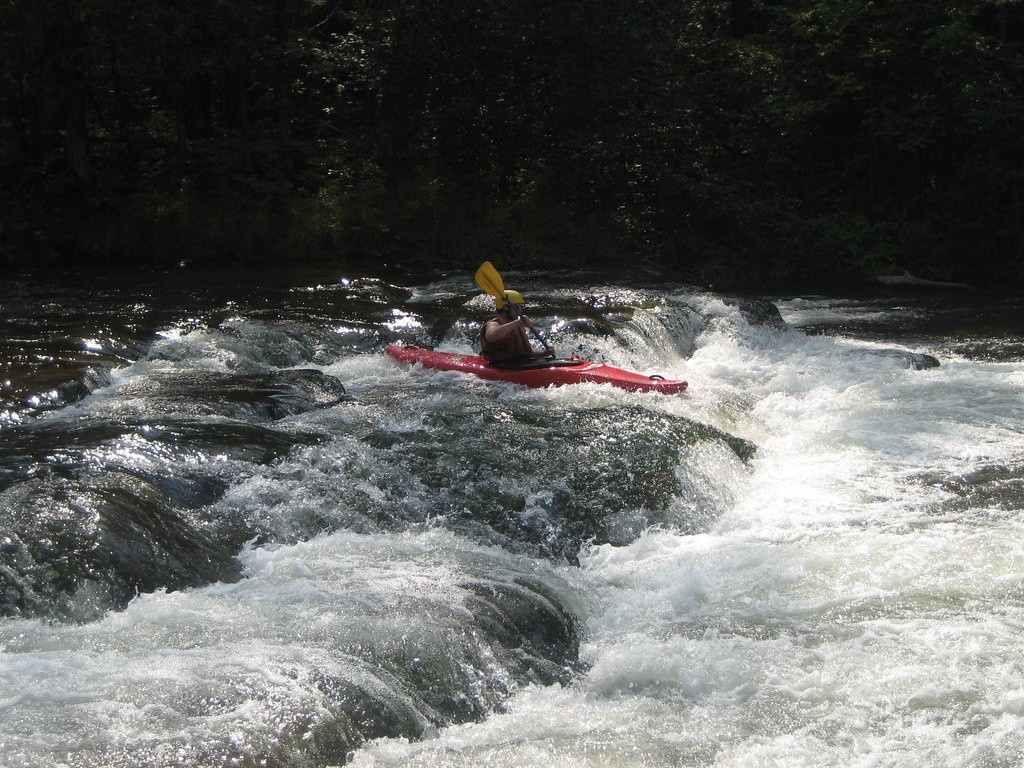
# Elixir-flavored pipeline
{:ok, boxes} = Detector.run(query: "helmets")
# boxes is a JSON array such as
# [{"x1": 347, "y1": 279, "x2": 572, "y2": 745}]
[{"x1": 495, "y1": 290, "x2": 526, "y2": 309}]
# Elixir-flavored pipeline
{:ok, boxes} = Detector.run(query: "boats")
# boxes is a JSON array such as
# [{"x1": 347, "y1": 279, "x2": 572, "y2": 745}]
[{"x1": 384, "y1": 345, "x2": 688, "y2": 395}]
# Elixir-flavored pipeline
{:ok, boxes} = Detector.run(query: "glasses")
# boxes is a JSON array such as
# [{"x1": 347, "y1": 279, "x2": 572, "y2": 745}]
[{"x1": 517, "y1": 303, "x2": 525, "y2": 308}]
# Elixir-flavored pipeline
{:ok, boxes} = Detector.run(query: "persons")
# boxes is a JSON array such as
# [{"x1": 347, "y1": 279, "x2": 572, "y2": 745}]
[{"x1": 480, "y1": 290, "x2": 555, "y2": 369}]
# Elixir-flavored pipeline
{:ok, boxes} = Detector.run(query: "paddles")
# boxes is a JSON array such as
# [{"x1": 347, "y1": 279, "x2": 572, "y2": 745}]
[{"x1": 474, "y1": 261, "x2": 556, "y2": 358}]
